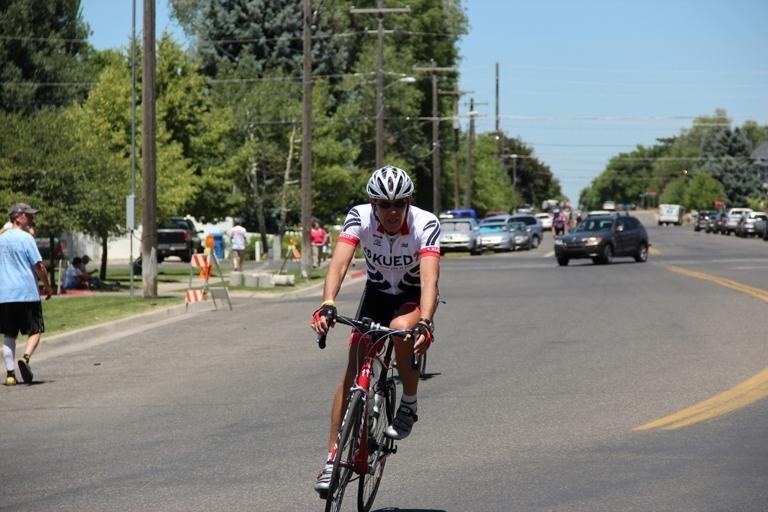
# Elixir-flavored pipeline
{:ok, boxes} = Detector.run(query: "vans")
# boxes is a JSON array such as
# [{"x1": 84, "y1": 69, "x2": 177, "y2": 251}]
[
  {"x1": 721, "y1": 207, "x2": 753, "y2": 235},
  {"x1": 480, "y1": 214, "x2": 543, "y2": 248},
  {"x1": 446, "y1": 208, "x2": 476, "y2": 224},
  {"x1": 35, "y1": 238, "x2": 63, "y2": 260},
  {"x1": 693, "y1": 210, "x2": 716, "y2": 231}
]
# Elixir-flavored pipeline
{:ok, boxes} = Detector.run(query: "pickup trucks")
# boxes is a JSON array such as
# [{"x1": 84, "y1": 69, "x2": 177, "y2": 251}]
[{"x1": 155, "y1": 217, "x2": 204, "y2": 263}]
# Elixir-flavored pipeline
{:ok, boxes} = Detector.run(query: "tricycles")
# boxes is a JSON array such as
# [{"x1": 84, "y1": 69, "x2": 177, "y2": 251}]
[{"x1": 657, "y1": 203, "x2": 684, "y2": 225}]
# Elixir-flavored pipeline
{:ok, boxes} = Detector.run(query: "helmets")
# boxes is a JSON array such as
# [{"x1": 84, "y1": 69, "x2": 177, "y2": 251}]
[{"x1": 366, "y1": 166, "x2": 415, "y2": 200}]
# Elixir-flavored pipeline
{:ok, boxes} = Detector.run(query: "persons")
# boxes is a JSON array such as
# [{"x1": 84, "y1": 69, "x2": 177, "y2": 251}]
[
  {"x1": 309, "y1": 219, "x2": 325, "y2": 268},
  {"x1": 63, "y1": 257, "x2": 114, "y2": 289},
  {"x1": 551, "y1": 208, "x2": 566, "y2": 236},
  {"x1": 0, "y1": 204, "x2": 52, "y2": 386},
  {"x1": 312, "y1": 166, "x2": 441, "y2": 498},
  {"x1": 75, "y1": 254, "x2": 114, "y2": 288},
  {"x1": 229, "y1": 217, "x2": 248, "y2": 272}
]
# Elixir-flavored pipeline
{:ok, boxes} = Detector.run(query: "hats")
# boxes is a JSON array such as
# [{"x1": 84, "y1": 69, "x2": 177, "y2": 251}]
[{"x1": 8, "y1": 202, "x2": 43, "y2": 216}]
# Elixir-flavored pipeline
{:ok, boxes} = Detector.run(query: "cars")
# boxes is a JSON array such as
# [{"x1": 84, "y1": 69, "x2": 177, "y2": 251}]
[
  {"x1": 534, "y1": 212, "x2": 552, "y2": 229},
  {"x1": 602, "y1": 201, "x2": 615, "y2": 210},
  {"x1": 735, "y1": 212, "x2": 767, "y2": 238},
  {"x1": 706, "y1": 213, "x2": 718, "y2": 234},
  {"x1": 512, "y1": 203, "x2": 534, "y2": 214},
  {"x1": 508, "y1": 221, "x2": 533, "y2": 251},
  {"x1": 475, "y1": 222, "x2": 514, "y2": 251},
  {"x1": 714, "y1": 212, "x2": 727, "y2": 234}
]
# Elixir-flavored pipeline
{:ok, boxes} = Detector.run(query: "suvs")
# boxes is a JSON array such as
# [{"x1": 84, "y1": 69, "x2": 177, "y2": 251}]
[
  {"x1": 554, "y1": 212, "x2": 648, "y2": 265},
  {"x1": 439, "y1": 218, "x2": 476, "y2": 256}
]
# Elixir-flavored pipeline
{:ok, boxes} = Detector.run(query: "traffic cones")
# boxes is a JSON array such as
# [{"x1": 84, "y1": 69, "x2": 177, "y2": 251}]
[{"x1": 184, "y1": 251, "x2": 213, "y2": 305}]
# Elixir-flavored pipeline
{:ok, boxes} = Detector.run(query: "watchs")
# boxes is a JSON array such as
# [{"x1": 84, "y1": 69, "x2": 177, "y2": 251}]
[{"x1": 419, "y1": 317, "x2": 435, "y2": 332}]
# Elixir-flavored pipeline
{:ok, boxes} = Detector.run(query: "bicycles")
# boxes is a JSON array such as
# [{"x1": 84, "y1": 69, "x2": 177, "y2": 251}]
[{"x1": 314, "y1": 304, "x2": 442, "y2": 511}]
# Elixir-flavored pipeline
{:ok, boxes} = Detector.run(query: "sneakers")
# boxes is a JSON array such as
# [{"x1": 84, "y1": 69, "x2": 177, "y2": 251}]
[
  {"x1": 5, "y1": 370, "x2": 18, "y2": 386},
  {"x1": 387, "y1": 404, "x2": 419, "y2": 439},
  {"x1": 19, "y1": 354, "x2": 36, "y2": 384},
  {"x1": 315, "y1": 460, "x2": 346, "y2": 494}
]
